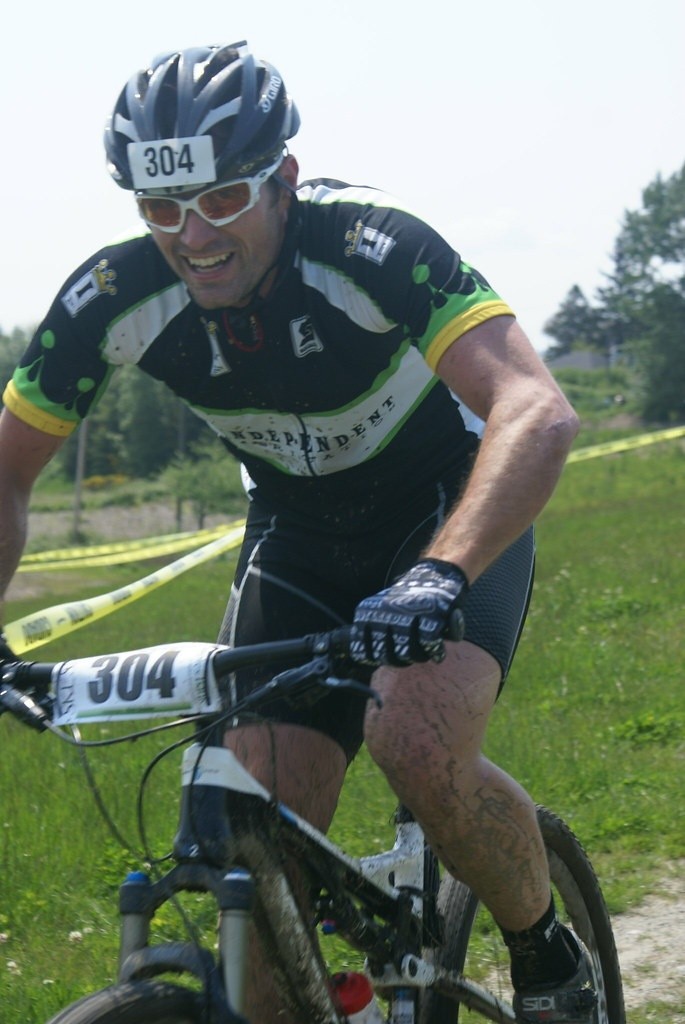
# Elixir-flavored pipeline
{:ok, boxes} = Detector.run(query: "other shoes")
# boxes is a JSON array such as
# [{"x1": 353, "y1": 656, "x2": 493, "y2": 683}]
[{"x1": 511, "y1": 923, "x2": 610, "y2": 1024}]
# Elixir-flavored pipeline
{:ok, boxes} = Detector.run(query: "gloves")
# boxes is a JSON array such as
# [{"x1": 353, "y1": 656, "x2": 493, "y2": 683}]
[{"x1": 349, "y1": 560, "x2": 468, "y2": 670}]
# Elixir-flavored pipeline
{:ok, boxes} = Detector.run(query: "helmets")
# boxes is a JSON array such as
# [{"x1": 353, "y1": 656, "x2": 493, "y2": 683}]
[{"x1": 101, "y1": 44, "x2": 302, "y2": 192}]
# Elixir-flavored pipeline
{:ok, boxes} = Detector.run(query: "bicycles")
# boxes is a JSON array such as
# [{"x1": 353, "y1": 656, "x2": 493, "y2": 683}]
[{"x1": 0, "y1": 623, "x2": 630, "y2": 1024}]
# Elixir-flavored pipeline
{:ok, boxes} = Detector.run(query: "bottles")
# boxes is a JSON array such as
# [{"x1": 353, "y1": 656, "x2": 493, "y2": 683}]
[{"x1": 333, "y1": 972, "x2": 387, "y2": 1024}]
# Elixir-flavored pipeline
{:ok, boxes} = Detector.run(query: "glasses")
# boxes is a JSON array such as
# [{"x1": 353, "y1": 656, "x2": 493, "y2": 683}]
[{"x1": 132, "y1": 150, "x2": 288, "y2": 236}]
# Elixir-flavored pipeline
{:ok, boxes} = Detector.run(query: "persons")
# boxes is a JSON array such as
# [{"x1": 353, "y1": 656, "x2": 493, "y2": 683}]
[{"x1": 1, "y1": 39, "x2": 601, "y2": 1024}]
